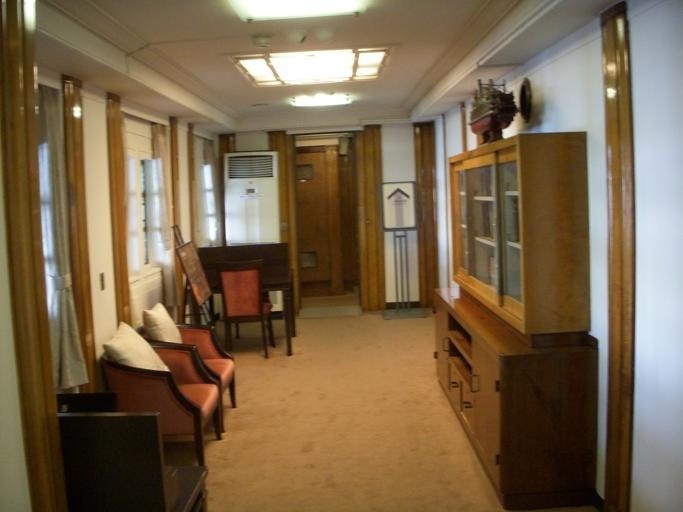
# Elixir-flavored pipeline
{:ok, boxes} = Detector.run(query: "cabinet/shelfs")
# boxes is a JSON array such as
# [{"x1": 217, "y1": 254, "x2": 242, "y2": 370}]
[
  {"x1": 449, "y1": 131, "x2": 591, "y2": 349},
  {"x1": 433, "y1": 294, "x2": 599, "y2": 510}
]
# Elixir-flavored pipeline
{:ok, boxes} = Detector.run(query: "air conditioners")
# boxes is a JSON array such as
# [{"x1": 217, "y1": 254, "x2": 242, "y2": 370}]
[{"x1": 223, "y1": 151, "x2": 281, "y2": 246}]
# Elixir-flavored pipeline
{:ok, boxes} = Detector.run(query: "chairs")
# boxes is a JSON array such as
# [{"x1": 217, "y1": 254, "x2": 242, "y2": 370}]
[
  {"x1": 217, "y1": 266, "x2": 275, "y2": 358},
  {"x1": 57, "y1": 410, "x2": 208, "y2": 512},
  {"x1": 100, "y1": 345, "x2": 221, "y2": 467},
  {"x1": 135, "y1": 324, "x2": 236, "y2": 432}
]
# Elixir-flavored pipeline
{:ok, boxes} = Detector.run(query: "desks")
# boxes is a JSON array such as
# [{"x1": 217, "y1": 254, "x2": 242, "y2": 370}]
[{"x1": 187, "y1": 264, "x2": 295, "y2": 356}]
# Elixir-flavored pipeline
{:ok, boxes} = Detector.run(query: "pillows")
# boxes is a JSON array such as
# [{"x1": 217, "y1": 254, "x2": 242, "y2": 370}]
[
  {"x1": 103, "y1": 321, "x2": 171, "y2": 373},
  {"x1": 144, "y1": 302, "x2": 182, "y2": 345}
]
